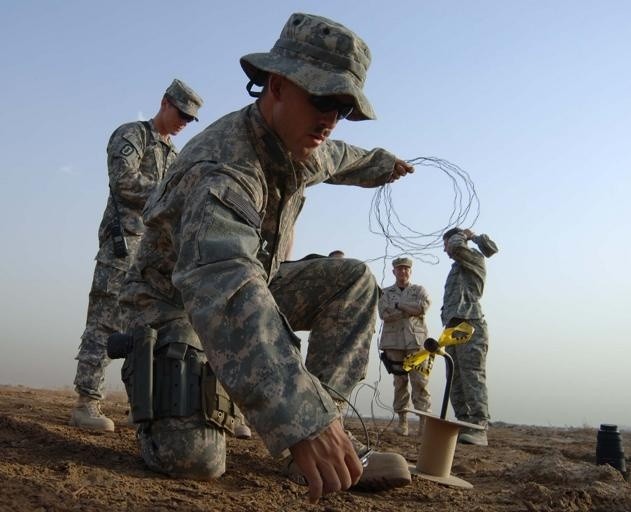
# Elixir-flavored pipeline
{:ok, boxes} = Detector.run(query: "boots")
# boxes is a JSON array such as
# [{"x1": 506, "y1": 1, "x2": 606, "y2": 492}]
[
  {"x1": 232, "y1": 420, "x2": 252, "y2": 437},
  {"x1": 396, "y1": 412, "x2": 489, "y2": 446},
  {"x1": 68, "y1": 395, "x2": 116, "y2": 434},
  {"x1": 285, "y1": 421, "x2": 412, "y2": 492}
]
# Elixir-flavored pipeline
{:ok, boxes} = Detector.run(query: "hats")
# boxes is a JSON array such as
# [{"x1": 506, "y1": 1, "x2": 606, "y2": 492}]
[
  {"x1": 392, "y1": 257, "x2": 413, "y2": 268},
  {"x1": 240, "y1": 13, "x2": 377, "y2": 122},
  {"x1": 164, "y1": 78, "x2": 202, "y2": 122},
  {"x1": 471, "y1": 232, "x2": 498, "y2": 258}
]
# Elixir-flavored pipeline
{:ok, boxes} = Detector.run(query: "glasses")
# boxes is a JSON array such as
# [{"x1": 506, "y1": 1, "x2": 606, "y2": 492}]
[
  {"x1": 309, "y1": 91, "x2": 355, "y2": 121},
  {"x1": 168, "y1": 99, "x2": 194, "y2": 122}
]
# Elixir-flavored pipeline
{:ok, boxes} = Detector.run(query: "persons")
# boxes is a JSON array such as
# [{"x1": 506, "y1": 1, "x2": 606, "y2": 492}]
[
  {"x1": 376, "y1": 255, "x2": 434, "y2": 437},
  {"x1": 439, "y1": 226, "x2": 491, "y2": 445},
  {"x1": 121, "y1": 12, "x2": 416, "y2": 503},
  {"x1": 67, "y1": 79, "x2": 204, "y2": 431}
]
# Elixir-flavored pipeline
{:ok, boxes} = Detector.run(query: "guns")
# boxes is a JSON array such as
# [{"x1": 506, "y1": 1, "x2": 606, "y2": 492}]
[{"x1": 107, "y1": 324, "x2": 158, "y2": 422}]
